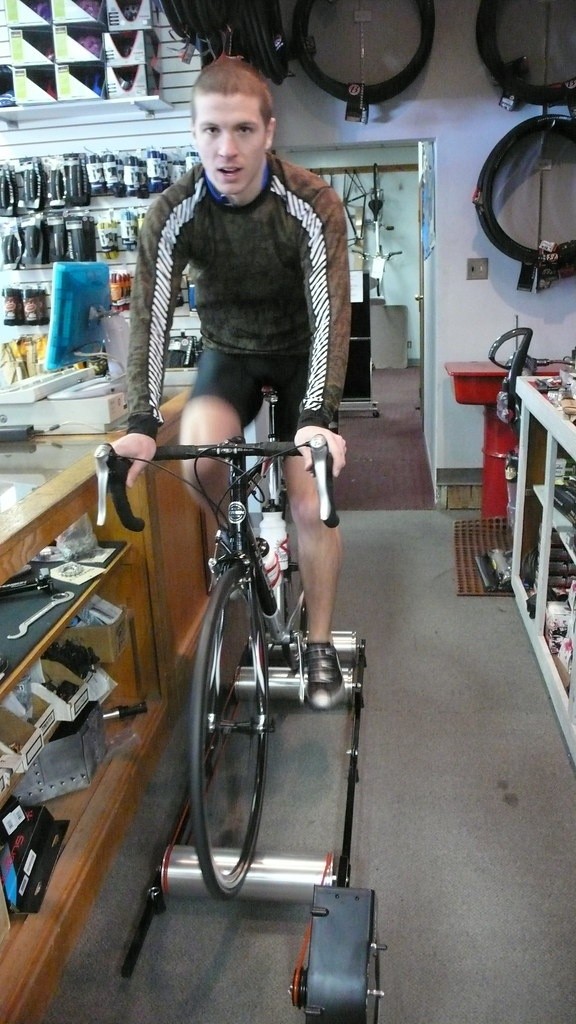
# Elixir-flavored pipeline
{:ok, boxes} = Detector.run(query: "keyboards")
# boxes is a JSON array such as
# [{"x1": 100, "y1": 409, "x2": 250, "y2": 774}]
[{"x1": 0, "y1": 367, "x2": 95, "y2": 405}]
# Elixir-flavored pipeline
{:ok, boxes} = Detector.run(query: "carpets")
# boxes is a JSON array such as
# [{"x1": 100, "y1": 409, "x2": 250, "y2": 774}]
[{"x1": 453, "y1": 516, "x2": 516, "y2": 598}]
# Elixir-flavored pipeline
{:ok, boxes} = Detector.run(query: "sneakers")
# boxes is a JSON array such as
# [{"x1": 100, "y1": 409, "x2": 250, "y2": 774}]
[
  {"x1": 230, "y1": 576, "x2": 247, "y2": 601},
  {"x1": 304, "y1": 642, "x2": 346, "y2": 708}
]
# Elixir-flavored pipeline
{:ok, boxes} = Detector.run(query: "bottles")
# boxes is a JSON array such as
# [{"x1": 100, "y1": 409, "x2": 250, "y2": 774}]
[
  {"x1": 256, "y1": 537, "x2": 282, "y2": 588},
  {"x1": 259, "y1": 499, "x2": 289, "y2": 570}
]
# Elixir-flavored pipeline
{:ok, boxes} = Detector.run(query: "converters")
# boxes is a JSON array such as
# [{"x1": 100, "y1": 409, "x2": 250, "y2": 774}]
[{"x1": 0, "y1": 425, "x2": 34, "y2": 442}]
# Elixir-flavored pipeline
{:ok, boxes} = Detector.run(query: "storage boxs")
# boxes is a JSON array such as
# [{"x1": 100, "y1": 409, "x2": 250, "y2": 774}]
[
  {"x1": 0, "y1": 0, "x2": 161, "y2": 108},
  {"x1": 0, "y1": 603, "x2": 129, "y2": 915}
]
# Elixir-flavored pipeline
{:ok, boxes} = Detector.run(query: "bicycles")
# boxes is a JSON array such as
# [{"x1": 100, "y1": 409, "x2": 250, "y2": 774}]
[{"x1": 92, "y1": 383, "x2": 342, "y2": 902}]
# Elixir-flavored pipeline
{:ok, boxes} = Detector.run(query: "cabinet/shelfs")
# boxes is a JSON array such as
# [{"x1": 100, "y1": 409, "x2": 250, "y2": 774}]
[
  {"x1": 0, "y1": 435, "x2": 171, "y2": 1024},
  {"x1": 510, "y1": 377, "x2": 576, "y2": 771}
]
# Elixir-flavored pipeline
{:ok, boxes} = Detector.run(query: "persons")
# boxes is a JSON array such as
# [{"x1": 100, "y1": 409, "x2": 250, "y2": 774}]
[{"x1": 109, "y1": 59, "x2": 351, "y2": 709}]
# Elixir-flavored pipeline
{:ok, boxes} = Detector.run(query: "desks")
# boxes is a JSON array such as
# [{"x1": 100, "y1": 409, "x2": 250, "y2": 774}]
[{"x1": 445, "y1": 358, "x2": 564, "y2": 516}]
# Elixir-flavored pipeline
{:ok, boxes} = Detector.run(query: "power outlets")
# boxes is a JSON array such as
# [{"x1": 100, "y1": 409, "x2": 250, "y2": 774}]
[{"x1": 466, "y1": 258, "x2": 489, "y2": 280}]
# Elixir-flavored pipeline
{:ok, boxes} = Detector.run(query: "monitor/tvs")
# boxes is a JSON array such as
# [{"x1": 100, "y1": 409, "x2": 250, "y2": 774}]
[{"x1": 44, "y1": 261, "x2": 131, "y2": 398}]
